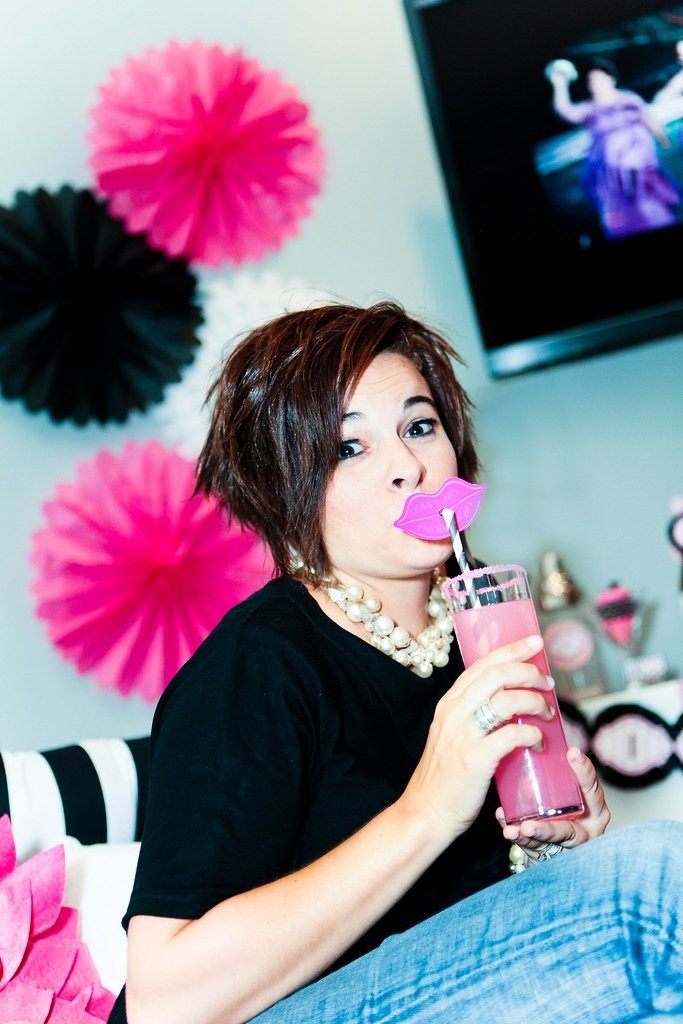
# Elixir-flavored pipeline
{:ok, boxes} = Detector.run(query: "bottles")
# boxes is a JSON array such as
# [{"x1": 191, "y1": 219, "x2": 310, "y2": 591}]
[{"x1": 534, "y1": 554, "x2": 607, "y2": 705}]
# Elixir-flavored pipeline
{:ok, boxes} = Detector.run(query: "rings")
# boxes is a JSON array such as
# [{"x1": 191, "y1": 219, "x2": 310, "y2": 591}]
[
  {"x1": 474, "y1": 702, "x2": 506, "y2": 733},
  {"x1": 537, "y1": 843, "x2": 563, "y2": 862}
]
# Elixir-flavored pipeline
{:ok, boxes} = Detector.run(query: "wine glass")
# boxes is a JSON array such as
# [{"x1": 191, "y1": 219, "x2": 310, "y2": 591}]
[{"x1": 591, "y1": 599, "x2": 651, "y2": 692}]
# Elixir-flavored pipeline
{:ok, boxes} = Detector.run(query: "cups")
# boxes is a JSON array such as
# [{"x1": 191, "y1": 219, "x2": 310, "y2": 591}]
[{"x1": 440, "y1": 566, "x2": 583, "y2": 825}]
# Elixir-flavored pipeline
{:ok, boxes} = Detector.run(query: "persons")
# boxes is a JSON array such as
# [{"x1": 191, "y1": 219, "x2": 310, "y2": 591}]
[
  {"x1": 548, "y1": 59, "x2": 683, "y2": 240},
  {"x1": 109, "y1": 298, "x2": 683, "y2": 1024}
]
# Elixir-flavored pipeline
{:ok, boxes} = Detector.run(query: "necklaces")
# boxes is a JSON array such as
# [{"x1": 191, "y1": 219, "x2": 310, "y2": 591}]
[{"x1": 298, "y1": 557, "x2": 454, "y2": 678}]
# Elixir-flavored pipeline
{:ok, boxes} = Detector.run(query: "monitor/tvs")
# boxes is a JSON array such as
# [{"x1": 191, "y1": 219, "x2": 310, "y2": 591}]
[{"x1": 403, "y1": 0, "x2": 683, "y2": 379}]
